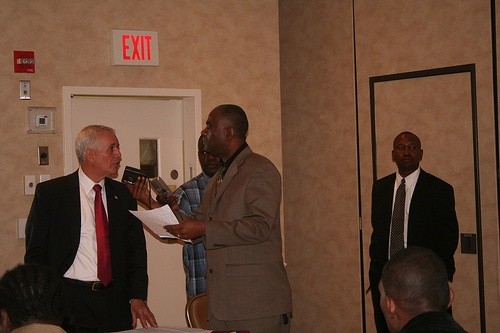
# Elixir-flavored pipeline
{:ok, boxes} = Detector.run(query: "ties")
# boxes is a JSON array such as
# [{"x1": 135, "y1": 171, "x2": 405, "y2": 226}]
[
  {"x1": 93, "y1": 184, "x2": 112, "y2": 288},
  {"x1": 389, "y1": 178, "x2": 406, "y2": 258},
  {"x1": 215, "y1": 166, "x2": 226, "y2": 195}
]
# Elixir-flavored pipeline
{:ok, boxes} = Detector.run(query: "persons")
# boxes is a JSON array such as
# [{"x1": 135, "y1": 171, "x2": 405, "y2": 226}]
[
  {"x1": 368, "y1": 132, "x2": 469, "y2": 333},
  {"x1": 24, "y1": 125, "x2": 158, "y2": 333},
  {"x1": 132, "y1": 104, "x2": 293, "y2": 333},
  {"x1": 0, "y1": 263, "x2": 67, "y2": 333}
]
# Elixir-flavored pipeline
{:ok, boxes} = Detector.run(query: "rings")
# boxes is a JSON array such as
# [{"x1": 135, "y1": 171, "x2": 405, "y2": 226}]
[{"x1": 177, "y1": 233, "x2": 180, "y2": 238}]
